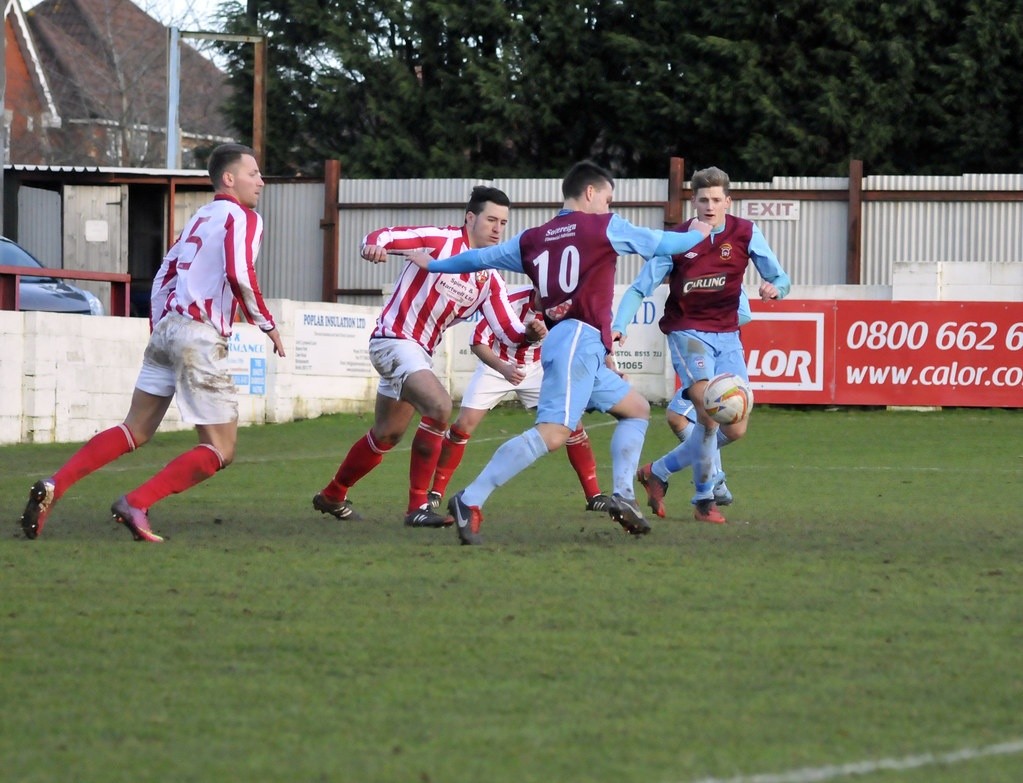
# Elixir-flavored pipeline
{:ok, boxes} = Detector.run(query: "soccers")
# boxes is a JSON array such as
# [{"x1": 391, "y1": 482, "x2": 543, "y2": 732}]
[{"x1": 702, "y1": 372, "x2": 755, "y2": 426}]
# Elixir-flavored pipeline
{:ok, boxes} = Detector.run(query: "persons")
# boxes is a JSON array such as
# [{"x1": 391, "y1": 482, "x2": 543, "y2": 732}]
[
  {"x1": 312, "y1": 186, "x2": 548, "y2": 526},
  {"x1": 427, "y1": 279, "x2": 610, "y2": 513},
  {"x1": 612, "y1": 167, "x2": 793, "y2": 523},
  {"x1": 405, "y1": 159, "x2": 714, "y2": 541},
  {"x1": 20, "y1": 144, "x2": 287, "y2": 542}
]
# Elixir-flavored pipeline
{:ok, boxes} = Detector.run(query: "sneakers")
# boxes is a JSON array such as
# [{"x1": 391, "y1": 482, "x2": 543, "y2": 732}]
[
  {"x1": 404, "y1": 503, "x2": 456, "y2": 527},
  {"x1": 691, "y1": 484, "x2": 733, "y2": 504},
  {"x1": 636, "y1": 462, "x2": 670, "y2": 518},
  {"x1": 584, "y1": 493, "x2": 612, "y2": 510},
  {"x1": 607, "y1": 493, "x2": 650, "y2": 535},
  {"x1": 312, "y1": 490, "x2": 360, "y2": 521},
  {"x1": 448, "y1": 489, "x2": 484, "y2": 544},
  {"x1": 694, "y1": 498, "x2": 727, "y2": 523},
  {"x1": 20, "y1": 480, "x2": 56, "y2": 538},
  {"x1": 111, "y1": 496, "x2": 165, "y2": 543},
  {"x1": 427, "y1": 490, "x2": 441, "y2": 508}
]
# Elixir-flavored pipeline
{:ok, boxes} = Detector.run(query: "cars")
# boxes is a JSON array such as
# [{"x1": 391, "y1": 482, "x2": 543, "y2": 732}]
[{"x1": 1, "y1": 239, "x2": 107, "y2": 317}]
